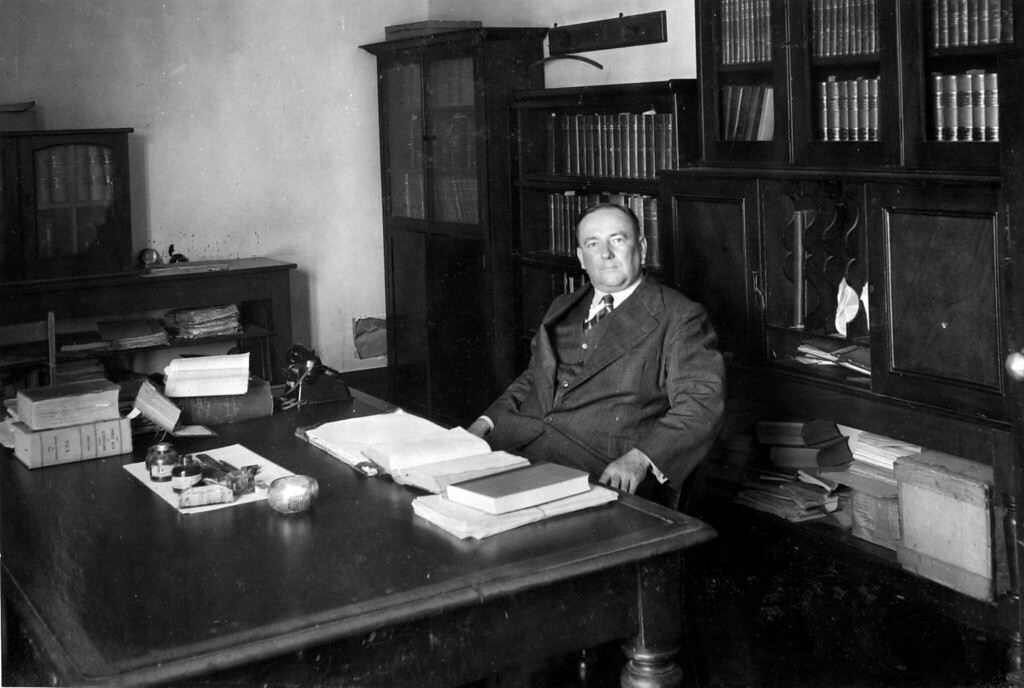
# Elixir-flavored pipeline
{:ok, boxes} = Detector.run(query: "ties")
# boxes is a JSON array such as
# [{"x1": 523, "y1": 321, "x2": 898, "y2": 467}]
[{"x1": 584, "y1": 294, "x2": 614, "y2": 335}]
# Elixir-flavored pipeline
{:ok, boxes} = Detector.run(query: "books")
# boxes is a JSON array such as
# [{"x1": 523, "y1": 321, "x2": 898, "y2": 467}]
[
  {"x1": 293, "y1": 405, "x2": 620, "y2": 541},
  {"x1": 148, "y1": 260, "x2": 229, "y2": 275},
  {"x1": 34, "y1": 143, "x2": 116, "y2": 259},
  {"x1": 390, "y1": 55, "x2": 482, "y2": 222},
  {"x1": 543, "y1": 106, "x2": 676, "y2": 307},
  {"x1": 718, "y1": 0, "x2": 1020, "y2": 143},
  {"x1": 0, "y1": 304, "x2": 275, "y2": 471},
  {"x1": 730, "y1": 412, "x2": 930, "y2": 523},
  {"x1": 787, "y1": 337, "x2": 872, "y2": 379}
]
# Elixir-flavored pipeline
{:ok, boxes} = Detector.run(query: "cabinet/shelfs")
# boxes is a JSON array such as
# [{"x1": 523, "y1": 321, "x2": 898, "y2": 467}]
[
  {"x1": 360, "y1": 0, "x2": 1024, "y2": 600},
  {"x1": 0, "y1": 124, "x2": 295, "y2": 393}
]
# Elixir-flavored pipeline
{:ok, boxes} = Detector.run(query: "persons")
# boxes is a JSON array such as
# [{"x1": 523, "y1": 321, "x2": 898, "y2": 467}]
[{"x1": 466, "y1": 201, "x2": 728, "y2": 495}]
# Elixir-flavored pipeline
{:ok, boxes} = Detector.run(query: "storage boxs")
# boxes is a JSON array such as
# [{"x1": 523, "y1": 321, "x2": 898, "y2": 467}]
[{"x1": 894, "y1": 453, "x2": 993, "y2": 609}]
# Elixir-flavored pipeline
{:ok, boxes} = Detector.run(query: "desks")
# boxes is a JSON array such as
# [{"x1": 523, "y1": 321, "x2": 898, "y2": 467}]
[{"x1": 0, "y1": 379, "x2": 720, "y2": 688}]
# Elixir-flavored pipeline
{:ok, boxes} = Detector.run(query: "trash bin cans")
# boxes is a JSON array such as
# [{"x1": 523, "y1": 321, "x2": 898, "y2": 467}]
[{"x1": 279, "y1": 342, "x2": 353, "y2": 408}]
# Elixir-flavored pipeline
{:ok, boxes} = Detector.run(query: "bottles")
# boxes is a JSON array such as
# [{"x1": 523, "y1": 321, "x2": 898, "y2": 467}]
[
  {"x1": 149, "y1": 444, "x2": 177, "y2": 481},
  {"x1": 171, "y1": 455, "x2": 202, "y2": 493}
]
